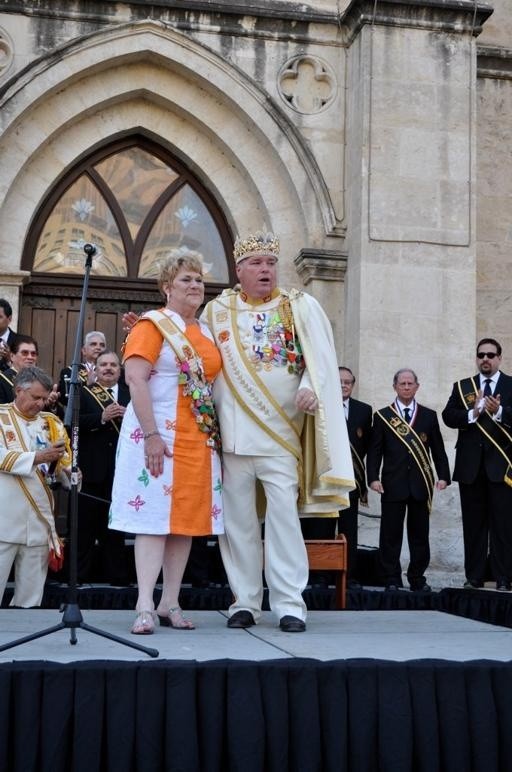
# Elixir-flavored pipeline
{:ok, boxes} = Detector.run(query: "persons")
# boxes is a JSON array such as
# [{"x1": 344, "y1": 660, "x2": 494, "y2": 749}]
[
  {"x1": 323, "y1": 367, "x2": 374, "y2": 588},
  {"x1": 0, "y1": 366, "x2": 83, "y2": 609},
  {"x1": 66, "y1": 348, "x2": 133, "y2": 586},
  {"x1": 105, "y1": 245, "x2": 222, "y2": 634},
  {"x1": 121, "y1": 229, "x2": 357, "y2": 635},
  {"x1": 57, "y1": 331, "x2": 108, "y2": 438},
  {"x1": 441, "y1": 337, "x2": 512, "y2": 591},
  {"x1": 0, "y1": 298, "x2": 20, "y2": 383},
  {"x1": 0, "y1": 334, "x2": 62, "y2": 413},
  {"x1": 366, "y1": 368, "x2": 452, "y2": 592}
]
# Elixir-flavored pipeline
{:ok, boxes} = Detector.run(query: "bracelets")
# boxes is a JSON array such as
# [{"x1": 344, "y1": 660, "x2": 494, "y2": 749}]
[{"x1": 143, "y1": 431, "x2": 160, "y2": 438}]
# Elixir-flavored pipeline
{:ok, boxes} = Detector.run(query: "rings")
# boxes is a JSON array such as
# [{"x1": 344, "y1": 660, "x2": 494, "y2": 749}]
[
  {"x1": 144, "y1": 456, "x2": 148, "y2": 460},
  {"x1": 310, "y1": 396, "x2": 315, "y2": 400}
]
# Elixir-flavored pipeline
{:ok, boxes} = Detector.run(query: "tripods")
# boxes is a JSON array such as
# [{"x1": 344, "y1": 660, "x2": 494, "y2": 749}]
[{"x1": 0, "y1": 385, "x2": 163, "y2": 658}]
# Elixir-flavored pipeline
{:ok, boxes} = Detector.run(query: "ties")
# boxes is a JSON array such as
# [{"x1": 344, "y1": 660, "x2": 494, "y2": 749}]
[
  {"x1": 107, "y1": 388, "x2": 114, "y2": 399},
  {"x1": 484, "y1": 379, "x2": 492, "y2": 397},
  {"x1": 0, "y1": 338, "x2": 2, "y2": 343},
  {"x1": 404, "y1": 408, "x2": 411, "y2": 424}
]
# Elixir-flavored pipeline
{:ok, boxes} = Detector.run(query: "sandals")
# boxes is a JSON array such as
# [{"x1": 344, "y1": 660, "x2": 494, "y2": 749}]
[
  {"x1": 131, "y1": 609, "x2": 156, "y2": 635},
  {"x1": 157, "y1": 606, "x2": 197, "y2": 630}
]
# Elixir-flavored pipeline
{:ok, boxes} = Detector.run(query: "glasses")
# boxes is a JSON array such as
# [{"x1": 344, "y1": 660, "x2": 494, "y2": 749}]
[
  {"x1": 16, "y1": 350, "x2": 39, "y2": 357},
  {"x1": 477, "y1": 352, "x2": 499, "y2": 359}
]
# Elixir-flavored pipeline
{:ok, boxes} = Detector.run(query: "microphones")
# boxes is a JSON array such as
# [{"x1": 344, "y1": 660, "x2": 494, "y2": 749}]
[
  {"x1": 84, "y1": 240, "x2": 103, "y2": 260},
  {"x1": 47, "y1": 437, "x2": 66, "y2": 477}
]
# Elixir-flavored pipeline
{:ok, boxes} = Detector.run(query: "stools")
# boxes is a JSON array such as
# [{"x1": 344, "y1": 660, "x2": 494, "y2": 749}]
[{"x1": 262, "y1": 533, "x2": 347, "y2": 610}]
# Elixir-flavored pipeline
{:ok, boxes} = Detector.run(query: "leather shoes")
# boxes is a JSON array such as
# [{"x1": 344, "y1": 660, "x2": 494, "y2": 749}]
[
  {"x1": 496, "y1": 580, "x2": 512, "y2": 591},
  {"x1": 409, "y1": 583, "x2": 432, "y2": 592},
  {"x1": 464, "y1": 579, "x2": 485, "y2": 588},
  {"x1": 348, "y1": 583, "x2": 363, "y2": 591},
  {"x1": 313, "y1": 583, "x2": 329, "y2": 589},
  {"x1": 386, "y1": 584, "x2": 398, "y2": 591},
  {"x1": 279, "y1": 615, "x2": 306, "y2": 632},
  {"x1": 227, "y1": 611, "x2": 254, "y2": 628}
]
